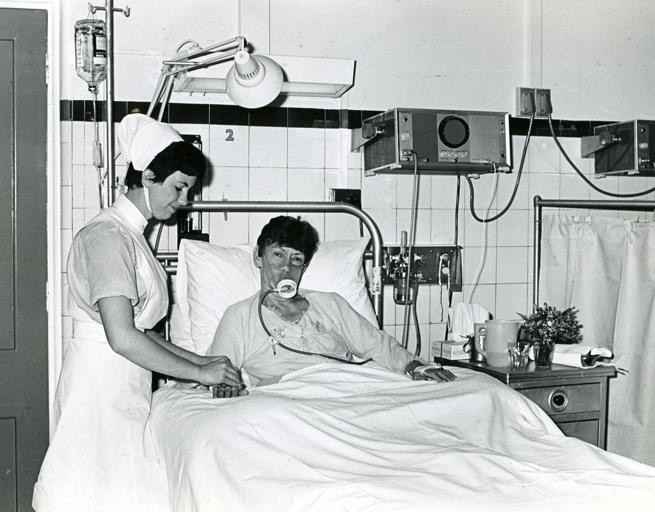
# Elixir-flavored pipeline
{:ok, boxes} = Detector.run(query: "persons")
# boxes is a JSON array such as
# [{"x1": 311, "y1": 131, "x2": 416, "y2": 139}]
[
  {"x1": 206, "y1": 211, "x2": 458, "y2": 407},
  {"x1": 52, "y1": 138, "x2": 245, "y2": 509}
]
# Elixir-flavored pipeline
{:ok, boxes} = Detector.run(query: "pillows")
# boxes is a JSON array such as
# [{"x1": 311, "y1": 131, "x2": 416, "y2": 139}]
[{"x1": 178, "y1": 238, "x2": 382, "y2": 389}]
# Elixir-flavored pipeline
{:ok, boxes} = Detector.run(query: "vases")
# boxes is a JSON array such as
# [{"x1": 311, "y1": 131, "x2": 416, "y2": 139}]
[{"x1": 533, "y1": 346, "x2": 553, "y2": 369}]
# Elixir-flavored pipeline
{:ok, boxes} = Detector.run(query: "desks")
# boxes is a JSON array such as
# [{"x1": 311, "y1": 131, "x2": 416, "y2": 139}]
[{"x1": 435, "y1": 356, "x2": 617, "y2": 451}]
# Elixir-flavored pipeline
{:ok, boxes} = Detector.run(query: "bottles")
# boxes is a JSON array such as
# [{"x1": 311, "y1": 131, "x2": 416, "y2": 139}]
[{"x1": 74, "y1": 19, "x2": 107, "y2": 91}]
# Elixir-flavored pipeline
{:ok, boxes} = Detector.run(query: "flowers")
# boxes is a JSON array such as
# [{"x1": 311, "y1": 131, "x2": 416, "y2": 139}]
[{"x1": 519, "y1": 304, "x2": 584, "y2": 360}]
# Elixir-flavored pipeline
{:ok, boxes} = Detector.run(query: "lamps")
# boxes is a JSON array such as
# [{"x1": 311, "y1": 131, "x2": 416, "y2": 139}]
[{"x1": 146, "y1": 34, "x2": 286, "y2": 121}]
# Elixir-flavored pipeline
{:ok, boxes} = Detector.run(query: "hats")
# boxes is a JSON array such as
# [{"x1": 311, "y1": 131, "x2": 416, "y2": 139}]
[{"x1": 116, "y1": 113, "x2": 185, "y2": 172}]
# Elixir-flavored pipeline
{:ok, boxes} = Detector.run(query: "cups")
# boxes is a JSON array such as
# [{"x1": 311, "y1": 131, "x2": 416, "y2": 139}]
[{"x1": 474, "y1": 320, "x2": 530, "y2": 372}]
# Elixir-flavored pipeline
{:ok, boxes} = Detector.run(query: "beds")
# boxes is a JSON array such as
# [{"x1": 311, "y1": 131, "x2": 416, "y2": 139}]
[{"x1": 150, "y1": 201, "x2": 655, "y2": 512}]
